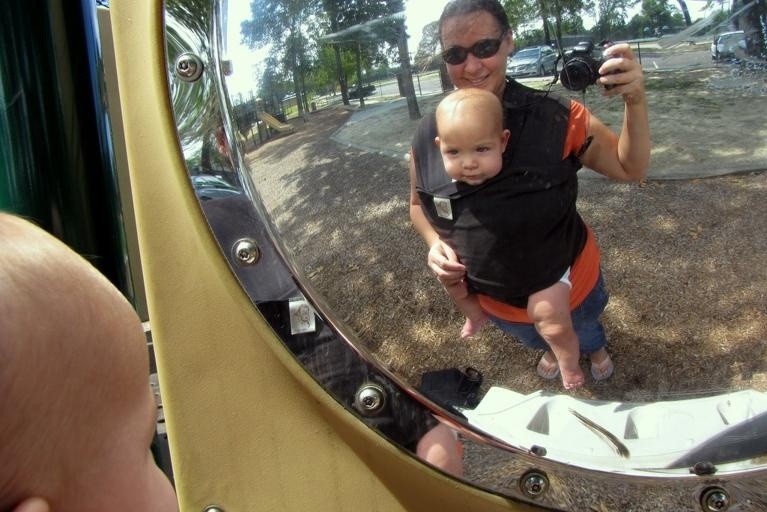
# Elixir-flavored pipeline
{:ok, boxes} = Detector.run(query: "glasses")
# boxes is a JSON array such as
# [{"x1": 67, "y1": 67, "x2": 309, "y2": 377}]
[{"x1": 439, "y1": 28, "x2": 508, "y2": 66}]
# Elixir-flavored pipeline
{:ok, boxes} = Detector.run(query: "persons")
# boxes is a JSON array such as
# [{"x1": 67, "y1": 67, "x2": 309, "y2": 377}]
[
  {"x1": 434, "y1": 87, "x2": 584, "y2": 391},
  {"x1": 406, "y1": 0, "x2": 650, "y2": 382},
  {"x1": 306, "y1": 326, "x2": 463, "y2": 480},
  {"x1": 1, "y1": 209, "x2": 177, "y2": 512}
]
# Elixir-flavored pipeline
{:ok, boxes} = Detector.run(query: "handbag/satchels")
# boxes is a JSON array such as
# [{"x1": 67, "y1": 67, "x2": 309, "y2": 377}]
[{"x1": 421, "y1": 368, "x2": 483, "y2": 415}]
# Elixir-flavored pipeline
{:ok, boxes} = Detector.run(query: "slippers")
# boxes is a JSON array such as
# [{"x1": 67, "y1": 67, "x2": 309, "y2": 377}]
[
  {"x1": 590, "y1": 347, "x2": 614, "y2": 381},
  {"x1": 536, "y1": 351, "x2": 559, "y2": 379}
]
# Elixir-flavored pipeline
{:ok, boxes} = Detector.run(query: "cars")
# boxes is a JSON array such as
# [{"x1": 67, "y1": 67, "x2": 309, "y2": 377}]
[
  {"x1": 711, "y1": 30, "x2": 746, "y2": 59},
  {"x1": 505, "y1": 45, "x2": 557, "y2": 77}
]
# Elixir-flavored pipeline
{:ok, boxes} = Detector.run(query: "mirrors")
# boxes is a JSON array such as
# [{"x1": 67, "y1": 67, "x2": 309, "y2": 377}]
[{"x1": 106, "y1": 0, "x2": 766, "y2": 511}]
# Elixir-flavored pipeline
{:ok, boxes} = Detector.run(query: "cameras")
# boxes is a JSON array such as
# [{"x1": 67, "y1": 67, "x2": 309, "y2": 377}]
[{"x1": 560, "y1": 38, "x2": 617, "y2": 91}]
